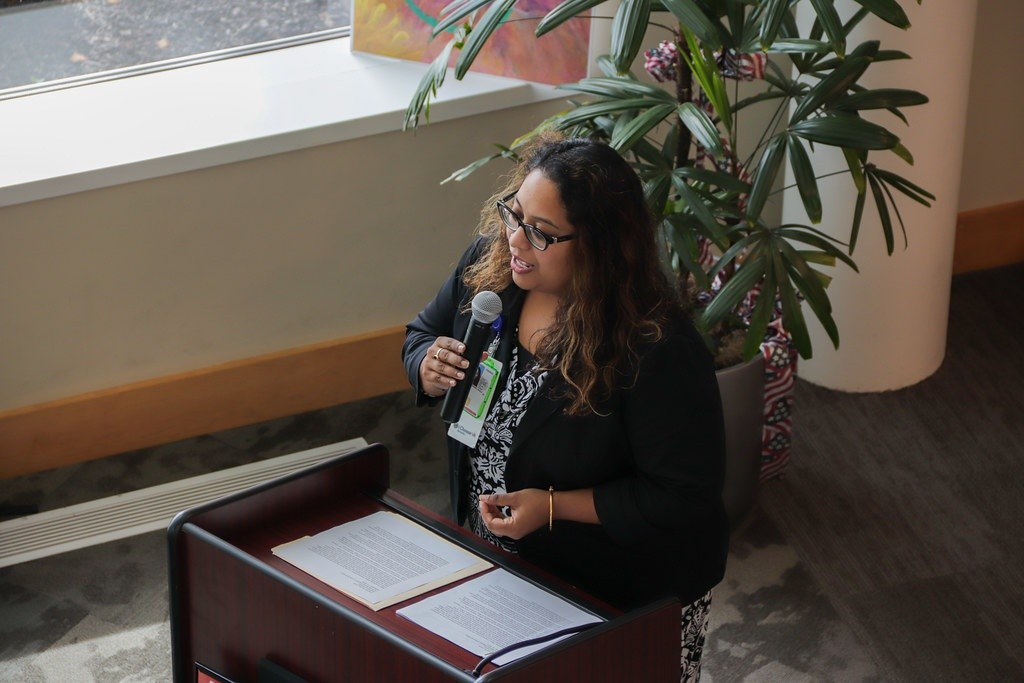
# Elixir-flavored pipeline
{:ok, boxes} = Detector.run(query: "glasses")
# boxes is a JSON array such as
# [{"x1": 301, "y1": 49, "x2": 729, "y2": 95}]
[{"x1": 497, "y1": 190, "x2": 578, "y2": 251}]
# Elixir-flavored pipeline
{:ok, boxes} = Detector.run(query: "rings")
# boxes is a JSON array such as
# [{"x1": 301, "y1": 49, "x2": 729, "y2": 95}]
[{"x1": 433, "y1": 348, "x2": 443, "y2": 359}]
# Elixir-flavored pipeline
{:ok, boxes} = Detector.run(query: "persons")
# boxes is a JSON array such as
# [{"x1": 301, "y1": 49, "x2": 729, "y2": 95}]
[{"x1": 399, "y1": 138, "x2": 727, "y2": 683}]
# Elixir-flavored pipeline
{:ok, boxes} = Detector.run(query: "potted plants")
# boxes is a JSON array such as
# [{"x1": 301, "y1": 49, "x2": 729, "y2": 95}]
[{"x1": 402, "y1": 1, "x2": 937, "y2": 529}]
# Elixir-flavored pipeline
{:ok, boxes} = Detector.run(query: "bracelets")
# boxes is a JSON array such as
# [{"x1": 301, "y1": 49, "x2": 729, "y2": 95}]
[{"x1": 549, "y1": 486, "x2": 553, "y2": 532}]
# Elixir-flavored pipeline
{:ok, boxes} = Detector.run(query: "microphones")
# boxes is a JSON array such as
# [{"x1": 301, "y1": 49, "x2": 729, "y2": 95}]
[{"x1": 437, "y1": 290, "x2": 502, "y2": 424}]
